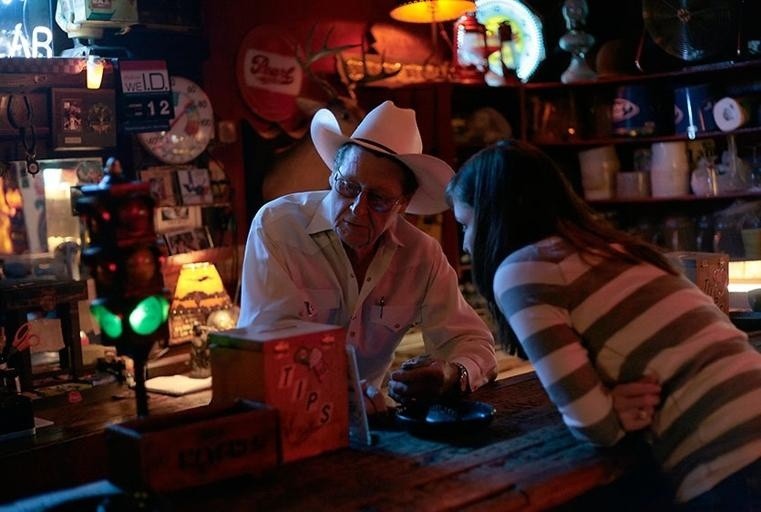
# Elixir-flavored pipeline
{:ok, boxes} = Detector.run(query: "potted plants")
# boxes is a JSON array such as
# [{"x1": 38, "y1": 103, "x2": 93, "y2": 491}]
[{"x1": 138, "y1": 169, "x2": 213, "y2": 255}]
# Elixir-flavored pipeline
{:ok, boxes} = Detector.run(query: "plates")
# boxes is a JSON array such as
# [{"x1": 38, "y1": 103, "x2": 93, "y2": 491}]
[{"x1": 372, "y1": 59, "x2": 761, "y2": 311}]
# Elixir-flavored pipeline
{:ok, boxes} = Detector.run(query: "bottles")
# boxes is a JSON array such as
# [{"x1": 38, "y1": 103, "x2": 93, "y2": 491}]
[{"x1": 308, "y1": 98, "x2": 459, "y2": 218}]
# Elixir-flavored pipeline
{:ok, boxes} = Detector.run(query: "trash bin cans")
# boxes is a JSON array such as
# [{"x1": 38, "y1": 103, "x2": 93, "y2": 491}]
[{"x1": 452, "y1": 361, "x2": 470, "y2": 395}]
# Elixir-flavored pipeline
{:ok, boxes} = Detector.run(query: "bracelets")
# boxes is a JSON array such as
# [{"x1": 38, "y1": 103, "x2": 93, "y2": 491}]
[{"x1": 395, "y1": 397, "x2": 497, "y2": 442}]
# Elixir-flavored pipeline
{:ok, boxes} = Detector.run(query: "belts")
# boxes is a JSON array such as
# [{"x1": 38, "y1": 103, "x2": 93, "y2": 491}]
[{"x1": 745, "y1": 144, "x2": 761, "y2": 191}]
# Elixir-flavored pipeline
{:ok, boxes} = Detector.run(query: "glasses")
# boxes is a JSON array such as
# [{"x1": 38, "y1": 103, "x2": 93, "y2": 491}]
[{"x1": 169, "y1": 262, "x2": 232, "y2": 378}]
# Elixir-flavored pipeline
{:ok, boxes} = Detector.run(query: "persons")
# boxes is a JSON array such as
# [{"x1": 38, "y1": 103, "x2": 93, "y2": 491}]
[
  {"x1": 237, "y1": 99, "x2": 498, "y2": 417},
  {"x1": 443, "y1": 137, "x2": 761, "y2": 512}
]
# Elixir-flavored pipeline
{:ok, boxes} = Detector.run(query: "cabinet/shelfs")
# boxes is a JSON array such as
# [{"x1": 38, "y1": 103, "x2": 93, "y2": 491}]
[{"x1": 703, "y1": 144, "x2": 720, "y2": 197}]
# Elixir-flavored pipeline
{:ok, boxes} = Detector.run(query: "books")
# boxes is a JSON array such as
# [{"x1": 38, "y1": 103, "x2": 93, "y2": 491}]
[{"x1": 207, "y1": 319, "x2": 350, "y2": 466}]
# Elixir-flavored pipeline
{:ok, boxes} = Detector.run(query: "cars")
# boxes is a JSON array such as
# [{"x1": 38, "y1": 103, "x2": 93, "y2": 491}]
[{"x1": 741, "y1": 228, "x2": 761, "y2": 259}]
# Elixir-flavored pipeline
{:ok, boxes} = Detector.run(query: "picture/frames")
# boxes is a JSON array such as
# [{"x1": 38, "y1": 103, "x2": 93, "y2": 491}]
[{"x1": 0, "y1": 369, "x2": 627, "y2": 512}]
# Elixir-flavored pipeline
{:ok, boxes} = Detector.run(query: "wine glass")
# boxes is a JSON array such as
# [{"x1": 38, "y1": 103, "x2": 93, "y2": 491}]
[
  {"x1": 409, "y1": 396, "x2": 419, "y2": 413},
  {"x1": 638, "y1": 407, "x2": 647, "y2": 421}
]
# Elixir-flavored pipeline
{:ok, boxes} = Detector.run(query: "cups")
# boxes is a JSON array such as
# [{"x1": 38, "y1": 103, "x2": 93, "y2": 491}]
[{"x1": 334, "y1": 174, "x2": 403, "y2": 213}]
